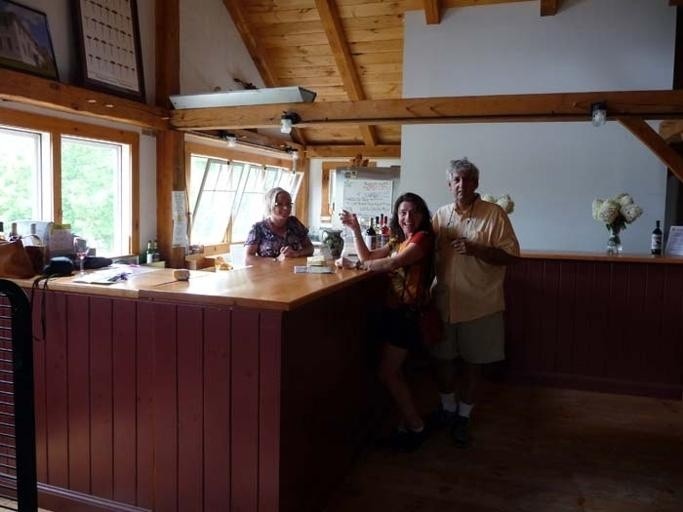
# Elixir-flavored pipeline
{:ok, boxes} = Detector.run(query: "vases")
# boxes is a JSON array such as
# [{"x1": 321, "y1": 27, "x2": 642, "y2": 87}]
[
  {"x1": 605, "y1": 226, "x2": 621, "y2": 255},
  {"x1": 322, "y1": 229, "x2": 344, "y2": 259}
]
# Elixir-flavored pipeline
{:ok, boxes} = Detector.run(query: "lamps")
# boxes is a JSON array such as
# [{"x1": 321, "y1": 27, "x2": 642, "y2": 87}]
[
  {"x1": 591, "y1": 102, "x2": 606, "y2": 127},
  {"x1": 169, "y1": 86, "x2": 318, "y2": 110},
  {"x1": 281, "y1": 110, "x2": 291, "y2": 134}
]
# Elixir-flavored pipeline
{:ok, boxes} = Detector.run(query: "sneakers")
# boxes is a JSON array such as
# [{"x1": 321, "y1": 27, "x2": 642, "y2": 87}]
[{"x1": 374, "y1": 399, "x2": 479, "y2": 454}]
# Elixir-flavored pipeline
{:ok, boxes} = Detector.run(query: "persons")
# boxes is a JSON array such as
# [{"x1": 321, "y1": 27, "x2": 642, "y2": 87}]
[
  {"x1": 432, "y1": 158, "x2": 520, "y2": 441},
  {"x1": 335, "y1": 192, "x2": 434, "y2": 454},
  {"x1": 246, "y1": 187, "x2": 314, "y2": 262}
]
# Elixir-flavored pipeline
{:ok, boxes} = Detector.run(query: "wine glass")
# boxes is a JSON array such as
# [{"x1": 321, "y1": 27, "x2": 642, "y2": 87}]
[{"x1": 73, "y1": 237, "x2": 92, "y2": 277}]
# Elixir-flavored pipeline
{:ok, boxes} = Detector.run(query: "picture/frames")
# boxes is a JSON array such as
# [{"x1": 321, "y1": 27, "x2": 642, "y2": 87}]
[
  {"x1": 0, "y1": 0, "x2": 60, "y2": 80},
  {"x1": 70, "y1": 0, "x2": 146, "y2": 103}
]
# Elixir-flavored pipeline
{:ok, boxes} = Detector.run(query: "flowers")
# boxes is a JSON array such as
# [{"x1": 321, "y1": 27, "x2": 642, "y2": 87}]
[
  {"x1": 592, "y1": 193, "x2": 643, "y2": 232},
  {"x1": 483, "y1": 194, "x2": 514, "y2": 213}
]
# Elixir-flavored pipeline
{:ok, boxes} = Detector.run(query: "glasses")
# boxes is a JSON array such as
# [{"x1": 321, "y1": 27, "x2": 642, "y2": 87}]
[
  {"x1": 446, "y1": 221, "x2": 470, "y2": 243},
  {"x1": 275, "y1": 202, "x2": 293, "y2": 207}
]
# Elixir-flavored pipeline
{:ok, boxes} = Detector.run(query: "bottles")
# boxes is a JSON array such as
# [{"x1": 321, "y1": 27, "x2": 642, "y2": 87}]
[
  {"x1": 145, "y1": 240, "x2": 160, "y2": 264},
  {"x1": 0, "y1": 221, "x2": 42, "y2": 248},
  {"x1": 366, "y1": 213, "x2": 390, "y2": 250},
  {"x1": 650, "y1": 220, "x2": 663, "y2": 254}
]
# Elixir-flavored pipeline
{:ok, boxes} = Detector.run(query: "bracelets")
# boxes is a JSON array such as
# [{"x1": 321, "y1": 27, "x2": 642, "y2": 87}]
[{"x1": 355, "y1": 260, "x2": 361, "y2": 268}]
